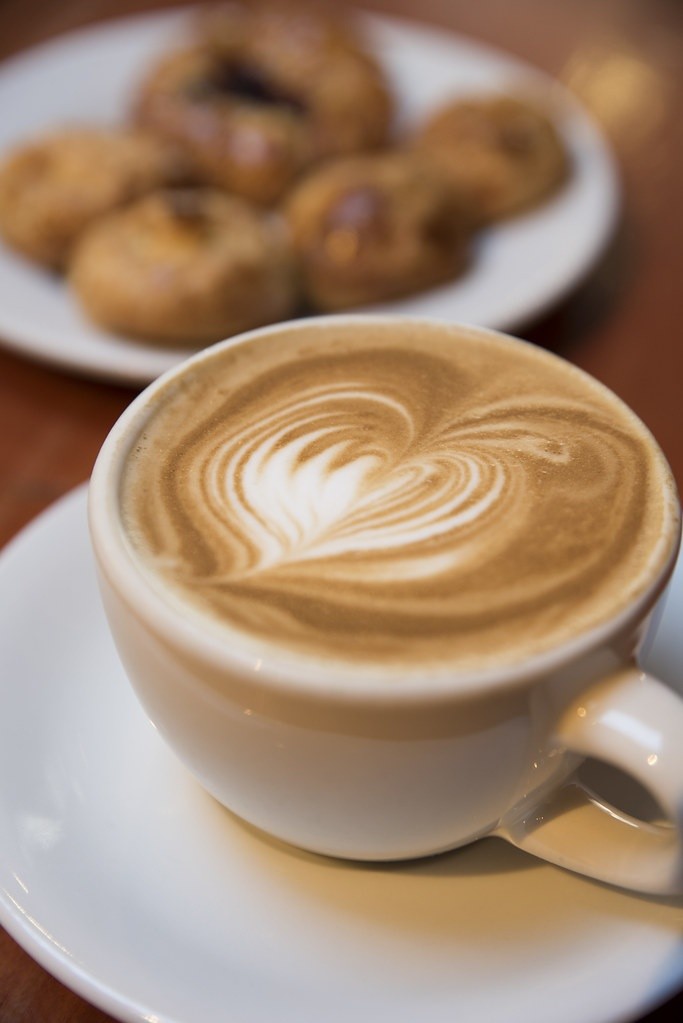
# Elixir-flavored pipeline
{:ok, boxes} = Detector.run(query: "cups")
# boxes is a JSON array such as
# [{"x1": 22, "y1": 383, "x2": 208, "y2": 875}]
[{"x1": 88, "y1": 311, "x2": 683, "y2": 897}]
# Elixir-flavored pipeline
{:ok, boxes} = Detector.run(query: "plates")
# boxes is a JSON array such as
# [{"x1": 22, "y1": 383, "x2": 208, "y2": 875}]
[
  {"x1": 0, "y1": 475, "x2": 683, "y2": 1023},
  {"x1": 0, "y1": 7, "x2": 622, "y2": 383}
]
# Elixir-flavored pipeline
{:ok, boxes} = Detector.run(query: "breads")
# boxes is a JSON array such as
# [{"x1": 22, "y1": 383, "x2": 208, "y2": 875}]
[{"x1": 0, "y1": 0, "x2": 570, "y2": 344}]
